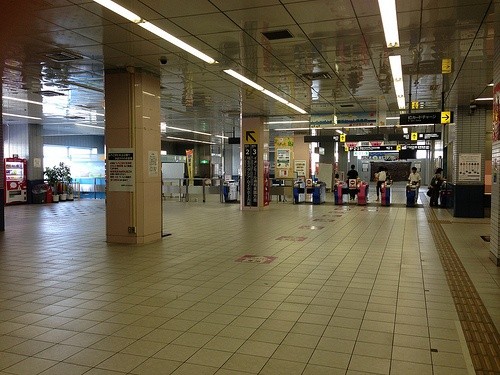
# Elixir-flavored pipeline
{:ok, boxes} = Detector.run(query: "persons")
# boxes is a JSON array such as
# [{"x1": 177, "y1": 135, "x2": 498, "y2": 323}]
[
  {"x1": 335, "y1": 174, "x2": 339, "y2": 182},
  {"x1": 347, "y1": 164, "x2": 358, "y2": 200},
  {"x1": 429, "y1": 168, "x2": 444, "y2": 208},
  {"x1": 375, "y1": 166, "x2": 387, "y2": 201},
  {"x1": 408, "y1": 167, "x2": 421, "y2": 202}
]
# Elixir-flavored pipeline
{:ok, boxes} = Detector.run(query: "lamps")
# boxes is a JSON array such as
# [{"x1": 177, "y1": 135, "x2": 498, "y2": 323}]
[
  {"x1": 224, "y1": 69, "x2": 309, "y2": 115},
  {"x1": 377, "y1": 0, "x2": 405, "y2": 110},
  {"x1": 92, "y1": 0, "x2": 219, "y2": 64}
]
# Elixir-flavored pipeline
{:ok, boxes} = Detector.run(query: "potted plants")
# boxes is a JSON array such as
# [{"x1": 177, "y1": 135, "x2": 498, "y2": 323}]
[{"x1": 43, "y1": 162, "x2": 75, "y2": 201}]
